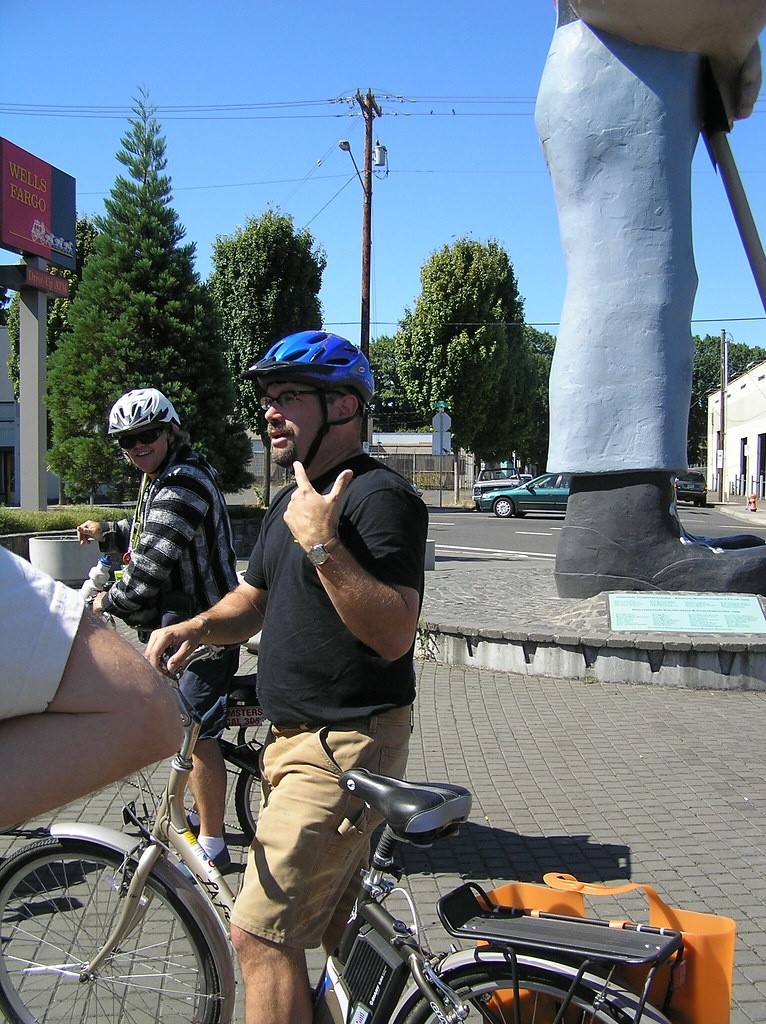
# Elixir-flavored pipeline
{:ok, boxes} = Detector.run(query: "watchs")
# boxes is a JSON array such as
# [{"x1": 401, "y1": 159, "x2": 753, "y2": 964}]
[{"x1": 307, "y1": 536, "x2": 340, "y2": 567}]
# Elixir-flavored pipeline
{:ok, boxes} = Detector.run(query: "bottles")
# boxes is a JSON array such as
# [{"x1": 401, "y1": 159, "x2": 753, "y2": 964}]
[{"x1": 82, "y1": 555, "x2": 112, "y2": 597}]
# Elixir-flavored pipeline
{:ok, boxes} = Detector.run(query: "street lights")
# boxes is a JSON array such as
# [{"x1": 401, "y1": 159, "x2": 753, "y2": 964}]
[{"x1": 338, "y1": 140, "x2": 370, "y2": 445}]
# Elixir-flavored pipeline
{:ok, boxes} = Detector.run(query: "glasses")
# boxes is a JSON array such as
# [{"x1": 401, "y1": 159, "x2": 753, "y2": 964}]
[
  {"x1": 260, "y1": 390, "x2": 345, "y2": 410},
  {"x1": 119, "y1": 425, "x2": 164, "y2": 449}
]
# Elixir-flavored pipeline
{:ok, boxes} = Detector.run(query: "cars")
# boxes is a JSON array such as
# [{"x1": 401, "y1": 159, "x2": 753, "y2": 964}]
[
  {"x1": 508, "y1": 474, "x2": 533, "y2": 483},
  {"x1": 480, "y1": 472, "x2": 570, "y2": 518}
]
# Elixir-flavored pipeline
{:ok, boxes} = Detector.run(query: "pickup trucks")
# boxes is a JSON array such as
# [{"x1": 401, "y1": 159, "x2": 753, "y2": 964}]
[
  {"x1": 474, "y1": 468, "x2": 523, "y2": 509},
  {"x1": 674, "y1": 472, "x2": 708, "y2": 507}
]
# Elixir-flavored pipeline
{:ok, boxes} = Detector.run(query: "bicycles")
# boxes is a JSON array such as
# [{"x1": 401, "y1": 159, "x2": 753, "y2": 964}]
[{"x1": 0, "y1": 526, "x2": 684, "y2": 1024}]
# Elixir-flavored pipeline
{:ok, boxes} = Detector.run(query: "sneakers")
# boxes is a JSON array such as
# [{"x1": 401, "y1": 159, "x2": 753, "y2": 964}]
[
  {"x1": 174, "y1": 843, "x2": 231, "y2": 883},
  {"x1": 186, "y1": 814, "x2": 225, "y2": 839}
]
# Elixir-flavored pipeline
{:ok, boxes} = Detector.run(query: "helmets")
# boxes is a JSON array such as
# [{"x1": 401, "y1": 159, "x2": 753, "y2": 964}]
[
  {"x1": 241, "y1": 331, "x2": 374, "y2": 404},
  {"x1": 107, "y1": 388, "x2": 181, "y2": 435}
]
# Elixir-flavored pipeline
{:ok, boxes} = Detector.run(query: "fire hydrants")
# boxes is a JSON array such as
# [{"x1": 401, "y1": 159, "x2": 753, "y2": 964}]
[{"x1": 749, "y1": 493, "x2": 757, "y2": 512}]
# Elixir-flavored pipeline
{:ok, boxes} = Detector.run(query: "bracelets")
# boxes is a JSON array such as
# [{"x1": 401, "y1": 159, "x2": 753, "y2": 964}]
[{"x1": 191, "y1": 616, "x2": 212, "y2": 640}]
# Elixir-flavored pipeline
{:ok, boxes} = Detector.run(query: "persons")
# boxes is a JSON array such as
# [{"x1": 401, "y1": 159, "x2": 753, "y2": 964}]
[
  {"x1": 0, "y1": 544, "x2": 183, "y2": 828},
  {"x1": 76, "y1": 387, "x2": 240, "y2": 884},
  {"x1": 145, "y1": 330, "x2": 429, "y2": 1023}
]
